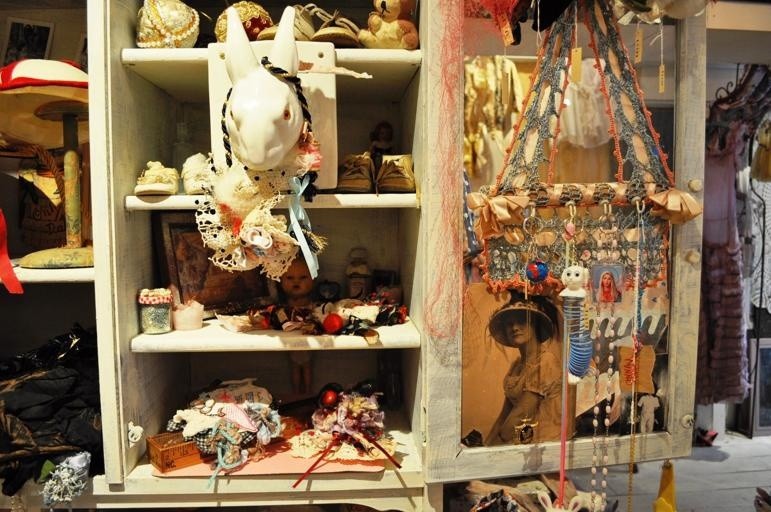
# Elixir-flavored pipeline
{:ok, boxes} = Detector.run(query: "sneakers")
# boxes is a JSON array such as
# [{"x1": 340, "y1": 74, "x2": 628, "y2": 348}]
[
  {"x1": 131, "y1": 153, "x2": 215, "y2": 196},
  {"x1": 337, "y1": 152, "x2": 374, "y2": 192},
  {"x1": 378, "y1": 152, "x2": 416, "y2": 192}
]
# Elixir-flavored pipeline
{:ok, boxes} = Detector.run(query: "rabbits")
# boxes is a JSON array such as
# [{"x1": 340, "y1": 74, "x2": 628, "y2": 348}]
[{"x1": 218, "y1": 1, "x2": 306, "y2": 172}]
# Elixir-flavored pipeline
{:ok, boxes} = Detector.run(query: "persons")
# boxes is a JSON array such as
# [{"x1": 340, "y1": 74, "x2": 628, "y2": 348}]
[
  {"x1": 479, "y1": 294, "x2": 577, "y2": 448},
  {"x1": 597, "y1": 271, "x2": 621, "y2": 302},
  {"x1": 280, "y1": 256, "x2": 316, "y2": 310}
]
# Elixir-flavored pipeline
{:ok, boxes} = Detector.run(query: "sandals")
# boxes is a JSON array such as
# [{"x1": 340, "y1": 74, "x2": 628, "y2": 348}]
[
  {"x1": 257, "y1": 4, "x2": 312, "y2": 49},
  {"x1": 313, "y1": 1, "x2": 361, "y2": 47}
]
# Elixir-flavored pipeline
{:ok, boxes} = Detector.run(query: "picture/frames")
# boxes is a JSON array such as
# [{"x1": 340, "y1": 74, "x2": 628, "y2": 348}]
[
  {"x1": 162, "y1": 211, "x2": 278, "y2": 319},
  {"x1": 1, "y1": 16, "x2": 55, "y2": 66}
]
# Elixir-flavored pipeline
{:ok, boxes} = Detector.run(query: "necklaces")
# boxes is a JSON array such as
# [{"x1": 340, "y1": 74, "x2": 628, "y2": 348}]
[{"x1": 587, "y1": 302, "x2": 615, "y2": 512}]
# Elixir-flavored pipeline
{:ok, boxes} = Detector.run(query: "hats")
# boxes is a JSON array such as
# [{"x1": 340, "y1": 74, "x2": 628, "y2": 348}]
[{"x1": 487, "y1": 298, "x2": 556, "y2": 348}]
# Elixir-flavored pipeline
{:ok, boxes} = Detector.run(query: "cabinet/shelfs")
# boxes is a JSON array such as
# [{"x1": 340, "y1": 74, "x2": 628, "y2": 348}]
[{"x1": 1, "y1": 0, "x2": 706, "y2": 512}]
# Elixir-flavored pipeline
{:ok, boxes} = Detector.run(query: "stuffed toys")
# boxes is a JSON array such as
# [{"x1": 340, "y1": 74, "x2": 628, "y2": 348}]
[
  {"x1": 356, "y1": 0, "x2": 418, "y2": 48},
  {"x1": 557, "y1": 260, "x2": 592, "y2": 385}
]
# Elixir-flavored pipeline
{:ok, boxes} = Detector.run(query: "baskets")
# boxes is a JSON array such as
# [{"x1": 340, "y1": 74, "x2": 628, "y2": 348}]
[{"x1": 145, "y1": 428, "x2": 219, "y2": 473}]
